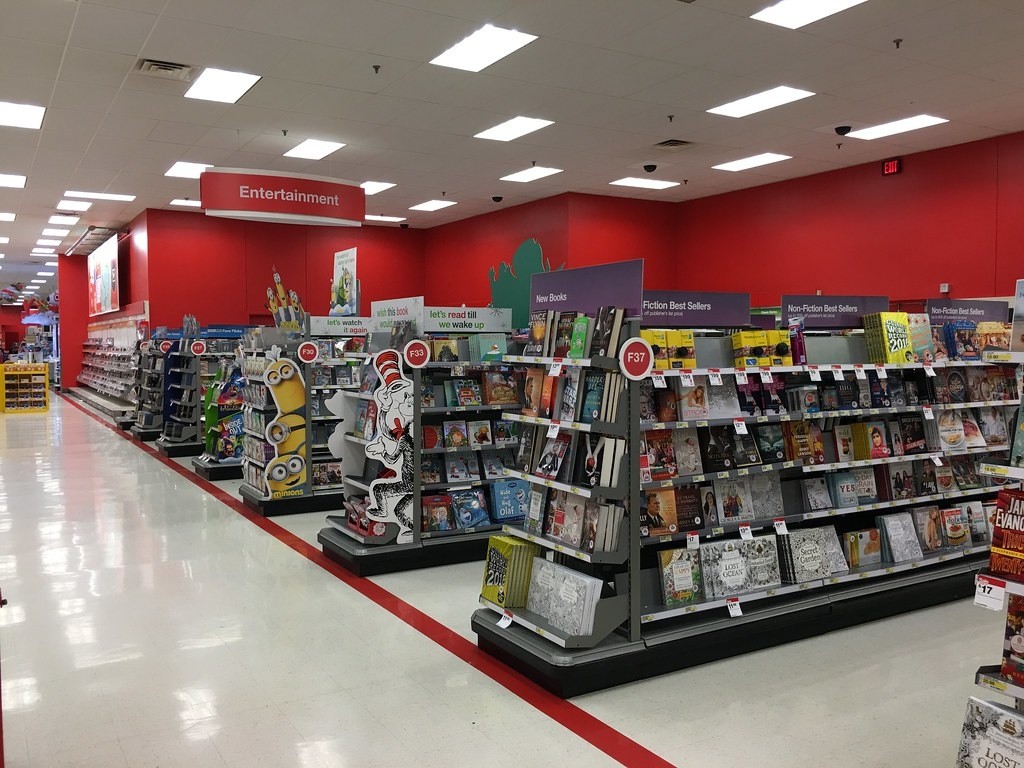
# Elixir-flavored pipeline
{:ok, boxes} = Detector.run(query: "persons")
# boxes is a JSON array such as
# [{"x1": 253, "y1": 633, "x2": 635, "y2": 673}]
[
  {"x1": 966, "y1": 505, "x2": 974, "y2": 534},
  {"x1": 984, "y1": 334, "x2": 1007, "y2": 351},
  {"x1": 570, "y1": 505, "x2": 581, "y2": 539},
  {"x1": 902, "y1": 470, "x2": 911, "y2": 488},
  {"x1": 920, "y1": 461, "x2": 938, "y2": 496},
  {"x1": 648, "y1": 444, "x2": 656, "y2": 458},
  {"x1": 9, "y1": 342, "x2": 18, "y2": 354},
  {"x1": 893, "y1": 471, "x2": 903, "y2": 496},
  {"x1": 924, "y1": 507, "x2": 943, "y2": 548},
  {"x1": 984, "y1": 407, "x2": 1006, "y2": 444},
  {"x1": 704, "y1": 491, "x2": 718, "y2": 524},
  {"x1": 894, "y1": 433, "x2": 904, "y2": 455},
  {"x1": 654, "y1": 441, "x2": 666, "y2": 461},
  {"x1": 679, "y1": 385, "x2": 705, "y2": 408},
  {"x1": 681, "y1": 437, "x2": 697, "y2": 470},
  {"x1": 641, "y1": 493, "x2": 666, "y2": 528},
  {"x1": 870, "y1": 426, "x2": 888, "y2": 458},
  {"x1": 931, "y1": 327, "x2": 949, "y2": 363}
]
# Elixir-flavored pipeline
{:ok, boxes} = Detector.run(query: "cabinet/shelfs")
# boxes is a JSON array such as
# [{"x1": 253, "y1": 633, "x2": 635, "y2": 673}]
[
  {"x1": 0, "y1": 323, "x2": 60, "y2": 414},
  {"x1": 69, "y1": 313, "x2": 1024, "y2": 768}
]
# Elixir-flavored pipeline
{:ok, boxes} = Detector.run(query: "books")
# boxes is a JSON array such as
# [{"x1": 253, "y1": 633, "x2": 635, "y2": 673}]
[
  {"x1": 481, "y1": 306, "x2": 630, "y2": 638},
  {"x1": 641, "y1": 311, "x2": 1012, "y2": 614},
  {"x1": 420, "y1": 333, "x2": 529, "y2": 532},
  {"x1": 311, "y1": 337, "x2": 365, "y2": 486},
  {"x1": 133, "y1": 337, "x2": 196, "y2": 438},
  {"x1": 199, "y1": 337, "x2": 238, "y2": 397},
  {"x1": 953, "y1": 278, "x2": 1024, "y2": 768},
  {"x1": 242, "y1": 358, "x2": 271, "y2": 493},
  {"x1": 353, "y1": 322, "x2": 412, "y2": 442}
]
